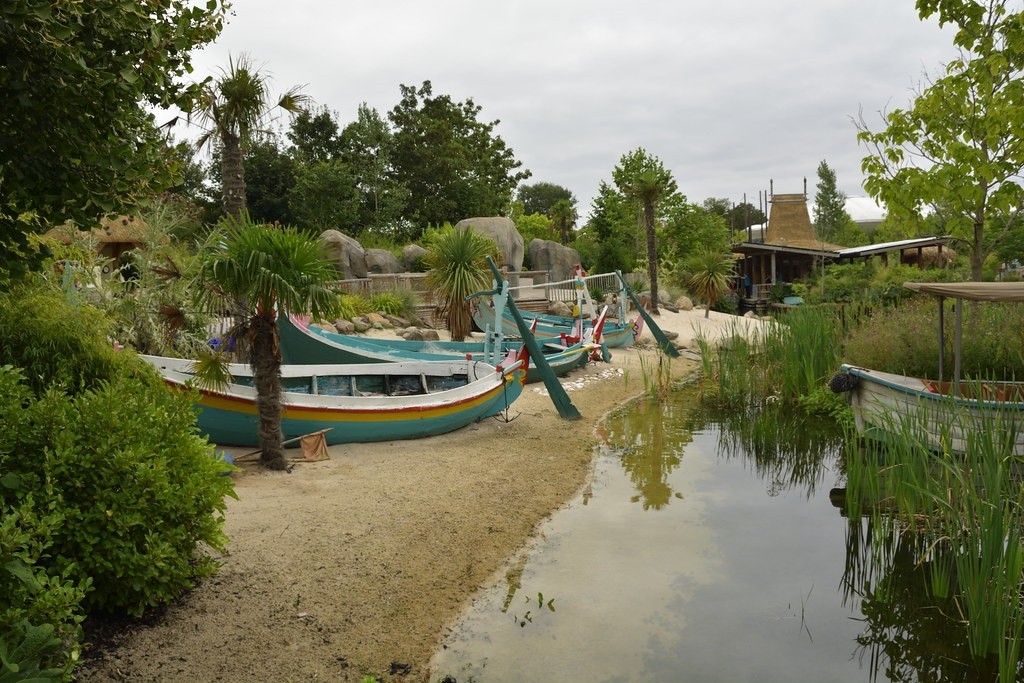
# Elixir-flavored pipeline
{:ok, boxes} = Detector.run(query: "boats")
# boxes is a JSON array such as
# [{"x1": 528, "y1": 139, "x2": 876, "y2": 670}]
[
  {"x1": 828, "y1": 446, "x2": 1023, "y2": 521},
  {"x1": 825, "y1": 278, "x2": 1024, "y2": 465},
  {"x1": 104, "y1": 314, "x2": 538, "y2": 450},
  {"x1": 467, "y1": 293, "x2": 648, "y2": 351},
  {"x1": 273, "y1": 293, "x2": 609, "y2": 384}
]
